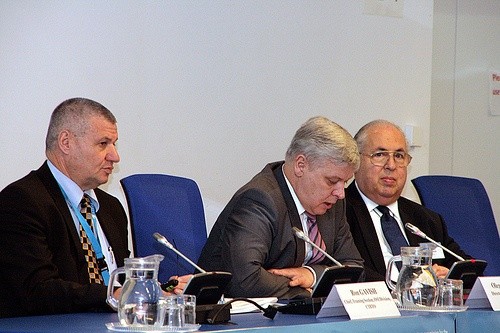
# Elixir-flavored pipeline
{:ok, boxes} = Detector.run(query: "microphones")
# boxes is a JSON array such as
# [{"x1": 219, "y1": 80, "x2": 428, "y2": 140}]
[
  {"x1": 152, "y1": 231, "x2": 206, "y2": 273},
  {"x1": 291, "y1": 226, "x2": 342, "y2": 266},
  {"x1": 405, "y1": 222, "x2": 465, "y2": 261}
]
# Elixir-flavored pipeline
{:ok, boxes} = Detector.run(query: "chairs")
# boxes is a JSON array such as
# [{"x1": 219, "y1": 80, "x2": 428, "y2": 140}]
[
  {"x1": 120, "y1": 174, "x2": 207, "y2": 283},
  {"x1": 411, "y1": 175, "x2": 500, "y2": 277}
]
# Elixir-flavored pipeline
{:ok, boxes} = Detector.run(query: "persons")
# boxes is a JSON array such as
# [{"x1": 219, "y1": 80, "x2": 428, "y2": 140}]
[
  {"x1": 0, "y1": 97, "x2": 193, "y2": 318},
  {"x1": 186, "y1": 117, "x2": 366, "y2": 306},
  {"x1": 346, "y1": 119, "x2": 472, "y2": 282}
]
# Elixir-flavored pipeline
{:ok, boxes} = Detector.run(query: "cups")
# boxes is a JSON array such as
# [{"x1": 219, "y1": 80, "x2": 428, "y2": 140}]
[
  {"x1": 155, "y1": 295, "x2": 196, "y2": 328},
  {"x1": 439, "y1": 279, "x2": 463, "y2": 307}
]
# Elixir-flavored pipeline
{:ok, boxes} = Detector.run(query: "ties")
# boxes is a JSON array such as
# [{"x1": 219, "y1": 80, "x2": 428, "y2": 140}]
[
  {"x1": 376, "y1": 205, "x2": 409, "y2": 271},
  {"x1": 304, "y1": 210, "x2": 326, "y2": 266},
  {"x1": 80, "y1": 197, "x2": 103, "y2": 285}
]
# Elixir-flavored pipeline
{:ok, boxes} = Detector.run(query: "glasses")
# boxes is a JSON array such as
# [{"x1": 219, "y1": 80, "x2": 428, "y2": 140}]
[{"x1": 360, "y1": 150, "x2": 412, "y2": 167}]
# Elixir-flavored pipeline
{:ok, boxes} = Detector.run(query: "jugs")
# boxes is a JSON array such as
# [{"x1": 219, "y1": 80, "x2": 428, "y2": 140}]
[
  {"x1": 107, "y1": 254, "x2": 165, "y2": 325},
  {"x1": 386, "y1": 242, "x2": 442, "y2": 310}
]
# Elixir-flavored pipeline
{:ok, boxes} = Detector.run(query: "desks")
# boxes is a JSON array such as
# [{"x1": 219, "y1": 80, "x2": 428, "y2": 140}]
[{"x1": 0, "y1": 290, "x2": 500, "y2": 333}]
